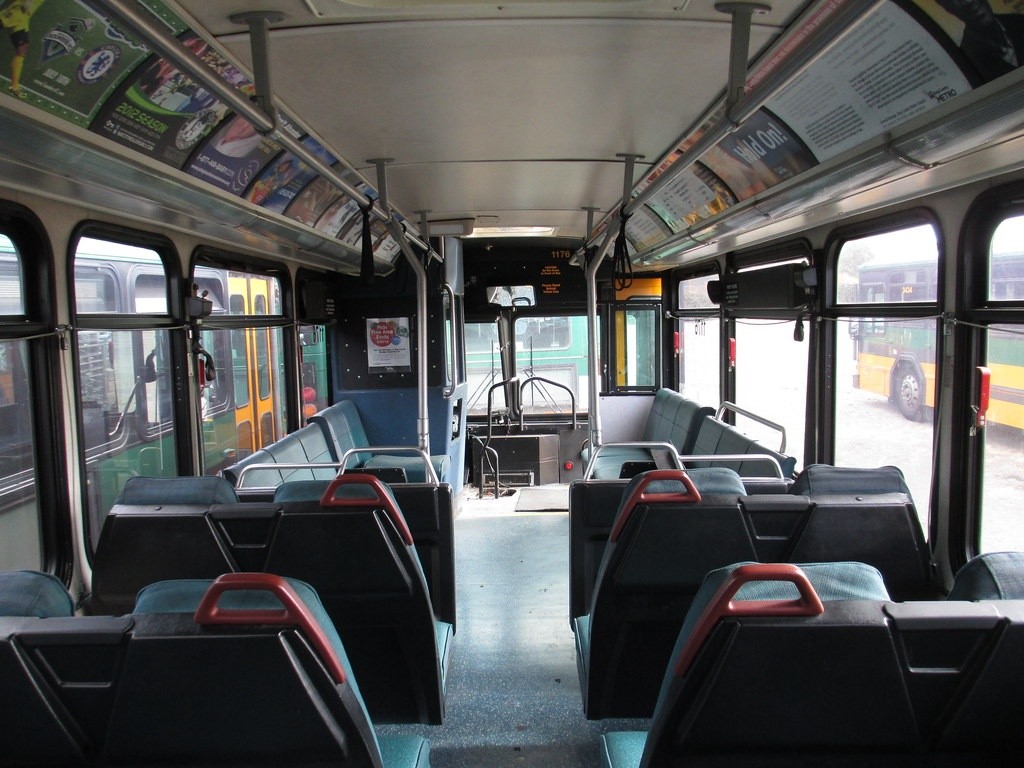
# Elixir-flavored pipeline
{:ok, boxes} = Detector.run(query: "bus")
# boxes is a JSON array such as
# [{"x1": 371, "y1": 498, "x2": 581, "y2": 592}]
[
  {"x1": 0, "y1": 245, "x2": 654, "y2": 570},
  {"x1": 848, "y1": 254, "x2": 1024, "y2": 430}
]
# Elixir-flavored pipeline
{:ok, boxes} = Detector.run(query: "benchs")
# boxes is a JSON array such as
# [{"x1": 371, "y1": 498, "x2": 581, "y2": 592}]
[
  {"x1": 307, "y1": 399, "x2": 448, "y2": 484},
  {"x1": 221, "y1": 422, "x2": 338, "y2": 491},
  {"x1": 581, "y1": 388, "x2": 717, "y2": 485},
  {"x1": 685, "y1": 402, "x2": 798, "y2": 477}
]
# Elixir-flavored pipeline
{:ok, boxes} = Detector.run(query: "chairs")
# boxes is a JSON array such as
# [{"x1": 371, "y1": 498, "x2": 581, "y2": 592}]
[{"x1": 0, "y1": 445, "x2": 1024, "y2": 768}]
